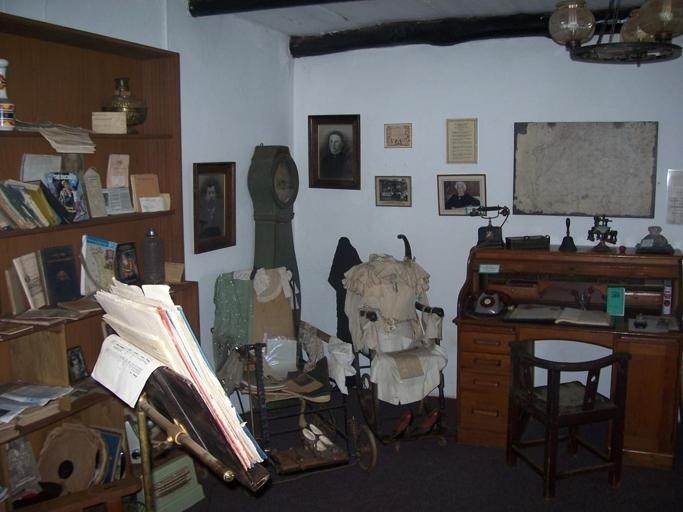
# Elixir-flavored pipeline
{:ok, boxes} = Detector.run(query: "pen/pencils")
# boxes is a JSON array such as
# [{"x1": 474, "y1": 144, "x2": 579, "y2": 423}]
[{"x1": 573, "y1": 290, "x2": 590, "y2": 311}]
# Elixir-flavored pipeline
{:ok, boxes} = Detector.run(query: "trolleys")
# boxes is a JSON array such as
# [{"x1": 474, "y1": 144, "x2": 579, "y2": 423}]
[{"x1": 337, "y1": 234, "x2": 445, "y2": 445}]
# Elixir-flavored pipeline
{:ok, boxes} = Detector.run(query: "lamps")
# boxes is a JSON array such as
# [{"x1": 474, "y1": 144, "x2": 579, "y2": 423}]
[{"x1": 548, "y1": 0, "x2": 683, "y2": 67}]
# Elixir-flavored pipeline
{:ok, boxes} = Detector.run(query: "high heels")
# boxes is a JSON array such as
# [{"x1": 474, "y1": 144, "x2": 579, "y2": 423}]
[
  {"x1": 385, "y1": 408, "x2": 413, "y2": 442},
  {"x1": 300, "y1": 429, "x2": 329, "y2": 458},
  {"x1": 412, "y1": 407, "x2": 441, "y2": 436},
  {"x1": 308, "y1": 424, "x2": 338, "y2": 451}
]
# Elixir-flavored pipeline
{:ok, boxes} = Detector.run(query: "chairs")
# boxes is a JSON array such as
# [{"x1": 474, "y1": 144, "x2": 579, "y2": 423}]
[
  {"x1": 507, "y1": 342, "x2": 632, "y2": 500},
  {"x1": 344, "y1": 235, "x2": 448, "y2": 470}
]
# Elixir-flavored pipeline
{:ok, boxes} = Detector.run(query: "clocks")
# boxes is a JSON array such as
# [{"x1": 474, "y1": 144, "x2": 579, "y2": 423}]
[{"x1": 247, "y1": 143, "x2": 302, "y2": 222}]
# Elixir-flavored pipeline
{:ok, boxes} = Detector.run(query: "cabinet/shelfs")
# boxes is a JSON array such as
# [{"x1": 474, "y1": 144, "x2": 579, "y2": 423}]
[
  {"x1": 0, "y1": 11, "x2": 199, "y2": 512},
  {"x1": 452, "y1": 245, "x2": 683, "y2": 471}
]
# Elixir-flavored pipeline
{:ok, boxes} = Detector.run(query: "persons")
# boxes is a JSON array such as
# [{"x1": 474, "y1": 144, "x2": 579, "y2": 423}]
[
  {"x1": 321, "y1": 132, "x2": 352, "y2": 180},
  {"x1": 198, "y1": 177, "x2": 224, "y2": 242},
  {"x1": 444, "y1": 182, "x2": 480, "y2": 210}
]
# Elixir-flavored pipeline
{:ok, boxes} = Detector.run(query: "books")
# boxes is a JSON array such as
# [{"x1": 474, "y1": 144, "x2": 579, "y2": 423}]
[
  {"x1": 45, "y1": 257, "x2": 85, "y2": 304},
  {"x1": 115, "y1": 241, "x2": 140, "y2": 283},
  {"x1": 56, "y1": 296, "x2": 102, "y2": 313},
  {"x1": 627, "y1": 318, "x2": 667, "y2": 332},
  {"x1": 0, "y1": 322, "x2": 34, "y2": 336},
  {"x1": 42, "y1": 245, "x2": 74, "y2": 262},
  {"x1": 15, "y1": 308, "x2": 89, "y2": 321},
  {"x1": 4, "y1": 266, "x2": 31, "y2": 315},
  {"x1": 240, "y1": 356, "x2": 331, "y2": 402},
  {"x1": 636, "y1": 314, "x2": 679, "y2": 331},
  {"x1": 38, "y1": 419, "x2": 127, "y2": 487},
  {"x1": 79, "y1": 235, "x2": 119, "y2": 295},
  {"x1": 607, "y1": 286, "x2": 626, "y2": 316},
  {"x1": 553, "y1": 306, "x2": 612, "y2": 328},
  {"x1": 0, "y1": 152, "x2": 171, "y2": 232},
  {"x1": 504, "y1": 301, "x2": 561, "y2": 323},
  {"x1": 88, "y1": 273, "x2": 268, "y2": 474},
  {"x1": 12, "y1": 117, "x2": 98, "y2": 153},
  {"x1": 1, "y1": 315, "x2": 65, "y2": 327},
  {"x1": 12, "y1": 249, "x2": 49, "y2": 309}
]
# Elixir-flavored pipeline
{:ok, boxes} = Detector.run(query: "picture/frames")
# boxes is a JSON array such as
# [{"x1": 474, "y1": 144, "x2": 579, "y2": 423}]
[
  {"x1": 375, "y1": 176, "x2": 412, "y2": 207},
  {"x1": 194, "y1": 161, "x2": 236, "y2": 254},
  {"x1": 383, "y1": 123, "x2": 412, "y2": 148},
  {"x1": 446, "y1": 118, "x2": 478, "y2": 163},
  {"x1": 307, "y1": 115, "x2": 361, "y2": 189},
  {"x1": 514, "y1": 121, "x2": 658, "y2": 218},
  {"x1": 437, "y1": 174, "x2": 487, "y2": 216}
]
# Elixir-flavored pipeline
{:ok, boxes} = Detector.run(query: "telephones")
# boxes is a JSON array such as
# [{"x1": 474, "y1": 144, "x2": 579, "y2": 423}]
[
  {"x1": 586, "y1": 214, "x2": 618, "y2": 253},
  {"x1": 469, "y1": 205, "x2": 510, "y2": 249},
  {"x1": 466, "y1": 288, "x2": 518, "y2": 317}
]
